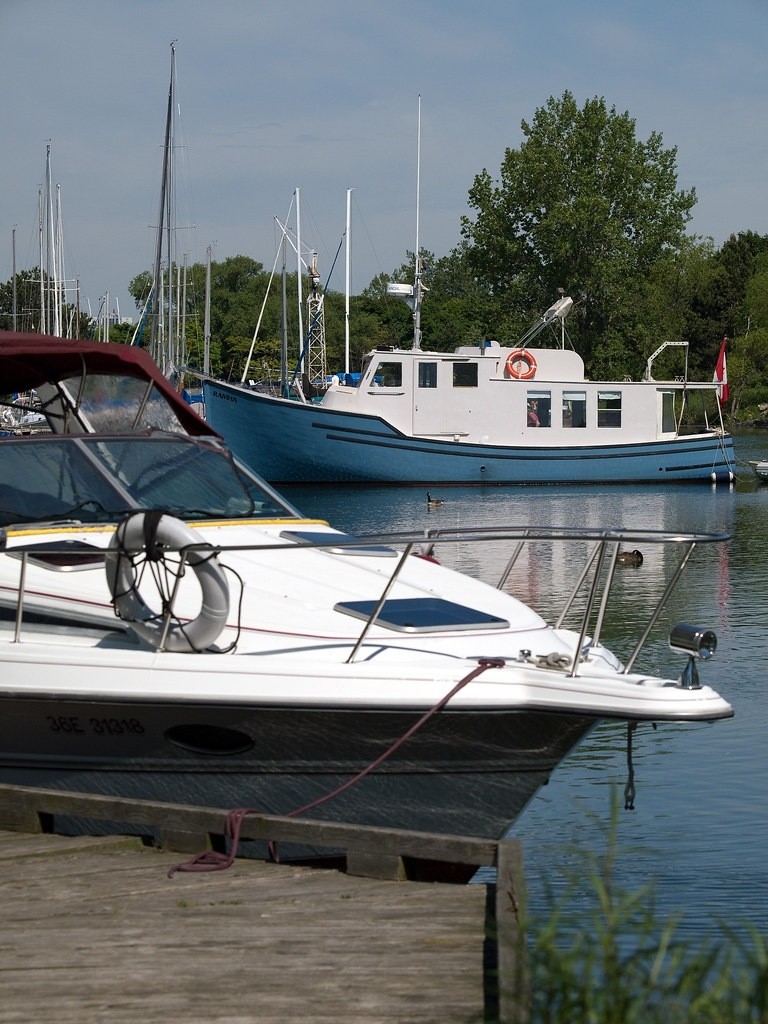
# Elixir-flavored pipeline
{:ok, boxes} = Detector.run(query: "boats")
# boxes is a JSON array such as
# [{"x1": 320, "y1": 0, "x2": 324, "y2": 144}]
[
  {"x1": 0, "y1": 332, "x2": 737, "y2": 886},
  {"x1": 749, "y1": 459, "x2": 768, "y2": 481}
]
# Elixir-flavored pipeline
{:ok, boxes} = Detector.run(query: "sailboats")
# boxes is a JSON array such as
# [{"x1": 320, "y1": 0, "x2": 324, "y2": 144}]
[
  {"x1": 1, "y1": 34, "x2": 387, "y2": 445},
  {"x1": 176, "y1": 89, "x2": 739, "y2": 489}
]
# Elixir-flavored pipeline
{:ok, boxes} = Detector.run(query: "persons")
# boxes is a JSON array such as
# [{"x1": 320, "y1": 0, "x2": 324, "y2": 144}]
[{"x1": 527, "y1": 409, "x2": 540, "y2": 427}]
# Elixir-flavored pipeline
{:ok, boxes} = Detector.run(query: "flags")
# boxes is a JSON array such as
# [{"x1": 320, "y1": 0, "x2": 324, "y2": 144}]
[{"x1": 712, "y1": 337, "x2": 729, "y2": 411}]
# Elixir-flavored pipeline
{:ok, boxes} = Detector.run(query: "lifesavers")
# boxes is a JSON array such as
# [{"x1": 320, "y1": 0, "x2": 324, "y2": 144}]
[
  {"x1": 101, "y1": 511, "x2": 233, "y2": 654},
  {"x1": 505, "y1": 350, "x2": 538, "y2": 379}
]
